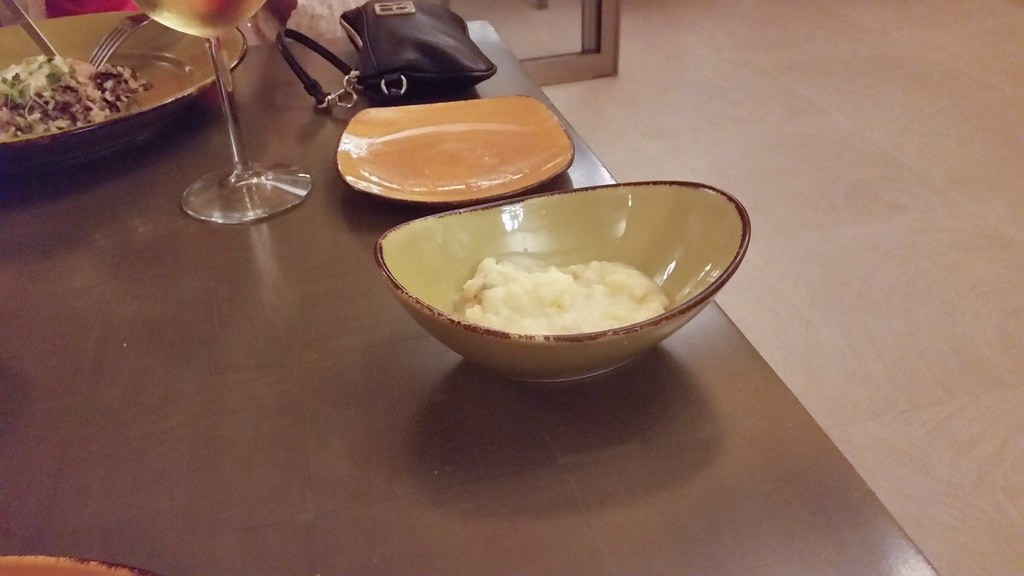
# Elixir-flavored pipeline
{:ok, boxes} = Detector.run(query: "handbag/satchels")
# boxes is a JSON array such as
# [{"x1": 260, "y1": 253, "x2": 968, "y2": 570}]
[{"x1": 278, "y1": 0, "x2": 497, "y2": 109}]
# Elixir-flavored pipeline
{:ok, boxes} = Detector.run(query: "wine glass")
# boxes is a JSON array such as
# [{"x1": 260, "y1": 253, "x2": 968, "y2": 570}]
[{"x1": 132, "y1": 1, "x2": 314, "y2": 224}]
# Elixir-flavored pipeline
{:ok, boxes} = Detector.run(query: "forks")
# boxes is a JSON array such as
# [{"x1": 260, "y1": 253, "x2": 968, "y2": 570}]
[{"x1": 91, "y1": 12, "x2": 154, "y2": 71}]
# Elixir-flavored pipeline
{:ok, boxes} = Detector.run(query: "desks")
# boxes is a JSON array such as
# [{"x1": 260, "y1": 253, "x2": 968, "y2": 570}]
[{"x1": 0, "y1": 0, "x2": 940, "y2": 576}]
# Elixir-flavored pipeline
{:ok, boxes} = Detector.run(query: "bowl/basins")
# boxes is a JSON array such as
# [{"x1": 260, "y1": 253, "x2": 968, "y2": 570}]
[{"x1": 375, "y1": 179, "x2": 751, "y2": 382}]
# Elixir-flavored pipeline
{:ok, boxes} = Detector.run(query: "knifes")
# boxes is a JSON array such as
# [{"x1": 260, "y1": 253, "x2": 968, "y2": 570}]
[{"x1": 0, "y1": 0, "x2": 58, "y2": 55}]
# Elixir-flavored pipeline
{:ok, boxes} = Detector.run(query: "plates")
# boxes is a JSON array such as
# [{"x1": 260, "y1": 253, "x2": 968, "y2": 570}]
[
  {"x1": 1, "y1": 15, "x2": 249, "y2": 170},
  {"x1": 335, "y1": 94, "x2": 573, "y2": 207}
]
